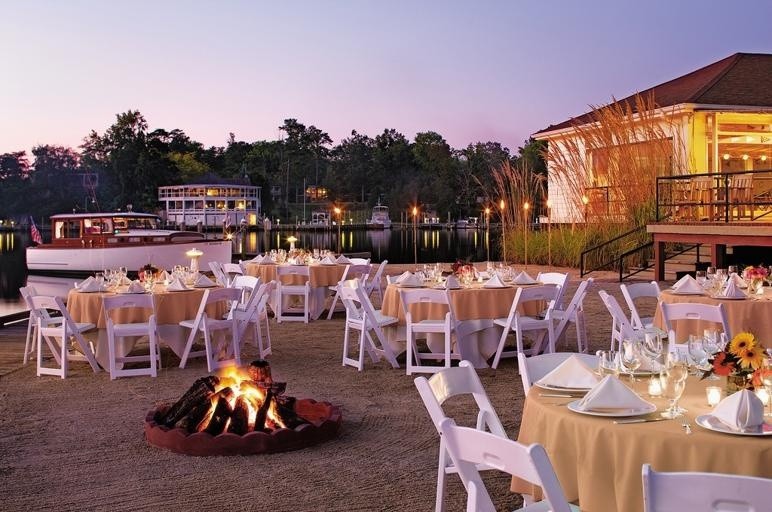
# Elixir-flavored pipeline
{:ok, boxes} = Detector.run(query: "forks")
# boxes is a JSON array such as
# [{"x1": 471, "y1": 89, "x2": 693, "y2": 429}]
[{"x1": 682, "y1": 420, "x2": 692, "y2": 435}]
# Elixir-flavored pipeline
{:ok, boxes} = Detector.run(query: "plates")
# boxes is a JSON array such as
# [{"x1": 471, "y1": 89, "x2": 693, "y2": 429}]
[
  {"x1": 673, "y1": 292, "x2": 705, "y2": 295},
  {"x1": 116, "y1": 291, "x2": 151, "y2": 294},
  {"x1": 397, "y1": 284, "x2": 428, "y2": 288},
  {"x1": 695, "y1": 413, "x2": 772, "y2": 436},
  {"x1": 567, "y1": 399, "x2": 658, "y2": 416},
  {"x1": 512, "y1": 282, "x2": 539, "y2": 285},
  {"x1": 534, "y1": 383, "x2": 591, "y2": 392},
  {"x1": 620, "y1": 370, "x2": 653, "y2": 377},
  {"x1": 482, "y1": 285, "x2": 513, "y2": 288},
  {"x1": 711, "y1": 296, "x2": 748, "y2": 300},
  {"x1": 433, "y1": 287, "x2": 465, "y2": 290},
  {"x1": 166, "y1": 289, "x2": 195, "y2": 292}
]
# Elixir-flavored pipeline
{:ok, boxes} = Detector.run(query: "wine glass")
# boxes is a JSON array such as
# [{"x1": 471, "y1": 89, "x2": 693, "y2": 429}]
[
  {"x1": 643, "y1": 334, "x2": 663, "y2": 375},
  {"x1": 104, "y1": 269, "x2": 110, "y2": 283},
  {"x1": 716, "y1": 269, "x2": 728, "y2": 282},
  {"x1": 314, "y1": 249, "x2": 319, "y2": 259},
  {"x1": 688, "y1": 334, "x2": 707, "y2": 376},
  {"x1": 598, "y1": 351, "x2": 619, "y2": 381},
  {"x1": 696, "y1": 271, "x2": 706, "y2": 287},
  {"x1": 703, "y1": 327, "x2": 723, "y2": 355},
  {"x1": 320, "y1": 250, "x2": 325, "y2": 259},
  {"x1": 325, "y1": 250, "x2": 330, "y2": 256},
  {"x1": 659, "y1": 370, "x2": 686, "y2": 420},
  {"x1": 761, "y1": 359, "x2": 772, "y2": 416},
  {"x1": 728, "y1": 266, "x2": 738, "y2": 277},
  {"x1": 281, "y1": 250, "x2": 286, "y2": 264},
  {"x1": 271, "y1": 249, "x2": 277, "y2": 256},
  {"x1": 278, "y1": 249, "x2": 283, "y2": 256},
  {"x1": 144, "y1": 270, "x2": 152, "y2": 282},
  {"x1": 96, "y1": 273, "x2": 104, "y2": 293},
  {"x1": 120, "y1": 267, "x2": 127, "y2": 277},
  {"x1": 665, "y1": 352, "x2": 688, "y2": 413},
  {"x1": 707, "y1": 267, "x2": 715, "y2": 279},
  {"x1": 621, "y1": 337, "x2": 642, "y2": 382}
]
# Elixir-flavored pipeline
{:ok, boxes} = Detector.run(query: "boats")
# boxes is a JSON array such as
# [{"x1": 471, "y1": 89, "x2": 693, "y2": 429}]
[
  {"x1": 26, "y1": 212, "x2": 232, "y2": 276},
  {"x1": 364, "y1": 196, "x2": 393, "y2": 230}
]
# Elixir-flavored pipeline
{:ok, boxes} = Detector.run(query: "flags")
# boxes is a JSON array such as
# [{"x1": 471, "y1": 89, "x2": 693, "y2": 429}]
[{"x1": 30, "y1": 216, "x2": 42, "y2": 245}]
